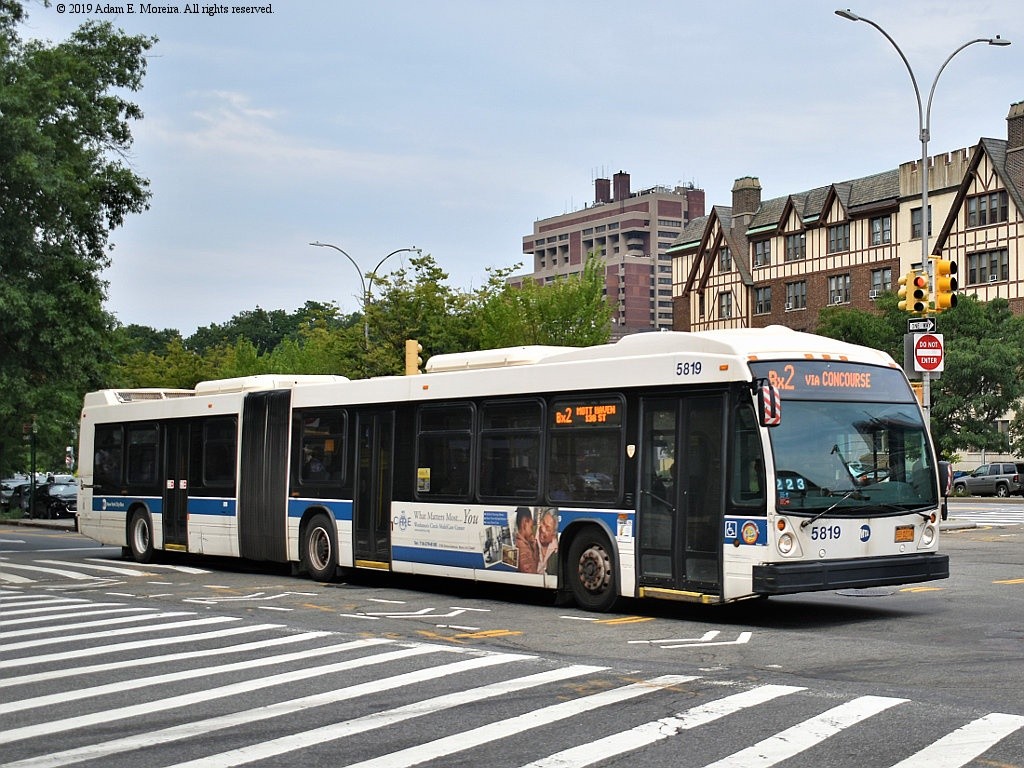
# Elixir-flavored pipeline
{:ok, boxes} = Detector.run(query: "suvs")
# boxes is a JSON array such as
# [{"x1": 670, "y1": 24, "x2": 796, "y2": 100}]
[{"x1": 953, "y1": 462, "x2": 1023, "y2": 498}]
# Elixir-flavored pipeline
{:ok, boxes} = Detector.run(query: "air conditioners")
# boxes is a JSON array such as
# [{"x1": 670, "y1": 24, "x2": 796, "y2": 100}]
[
  {"x1": 989, "y1": 274, "x2": 997, "y2": 282},
  {"x1": 869, "y1": 290, "x2": 881, "y2": 298},
  {"x1": 754, "y1": 261, "x2": 762, "y2": 266},
  {"x1": 784, "y1": 303, "x2": 792, "y2": 309},
  {"x1": 834, "y1": 296, "x2": 843, "y2": 303}
]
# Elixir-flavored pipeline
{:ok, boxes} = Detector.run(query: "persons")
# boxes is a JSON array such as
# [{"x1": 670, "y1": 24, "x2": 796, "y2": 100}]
[
  {"x1": 513, "y1": 507, "x2": 558, "y2": 575},
  {"x1": 305, "y1": 446, "x2": 326, "y2": 473}
]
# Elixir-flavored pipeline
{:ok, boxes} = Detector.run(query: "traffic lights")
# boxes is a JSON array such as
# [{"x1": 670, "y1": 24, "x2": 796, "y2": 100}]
[
  {"x1": 935, "y1": 258, "x2": 959, "y2": 311},
  {"x1": 404, "y1": 339, "x2": 424, "y2": 374},
  {"x1": 897, "y1": 272, "x2": 917, "y2": 313},
  {"x1": 912, "y1": 275, "x2": 930, "y2": 314}
]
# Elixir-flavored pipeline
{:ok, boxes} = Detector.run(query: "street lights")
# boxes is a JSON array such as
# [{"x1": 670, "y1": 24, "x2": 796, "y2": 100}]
[
  {"x1": 835, "y1": 7, "x2": 1014, "y2": 436},
  {"x1": 308, "y1": 240, "x2": 423, "y2": 350}
]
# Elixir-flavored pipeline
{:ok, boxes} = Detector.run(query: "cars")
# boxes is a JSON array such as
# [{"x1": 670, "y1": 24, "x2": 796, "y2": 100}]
[{"x1": 1, "y1": 471, "x2": 76, "y2": 519}]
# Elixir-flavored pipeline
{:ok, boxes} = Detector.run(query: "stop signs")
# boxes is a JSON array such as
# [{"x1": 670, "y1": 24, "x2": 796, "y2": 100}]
[{"x1": 912, "y1": 333, "x2": 945, "y2": 373}]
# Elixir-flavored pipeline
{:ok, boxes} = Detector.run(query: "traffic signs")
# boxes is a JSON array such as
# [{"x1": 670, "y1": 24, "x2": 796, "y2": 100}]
[{"x1": 906, "y1": 317, "x2": 937, "y2": 334}]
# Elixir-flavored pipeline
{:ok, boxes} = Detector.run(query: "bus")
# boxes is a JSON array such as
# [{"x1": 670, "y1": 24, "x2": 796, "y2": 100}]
[{"x1": 75, "y1": 321, "x2": 953, "y2": 616}]
